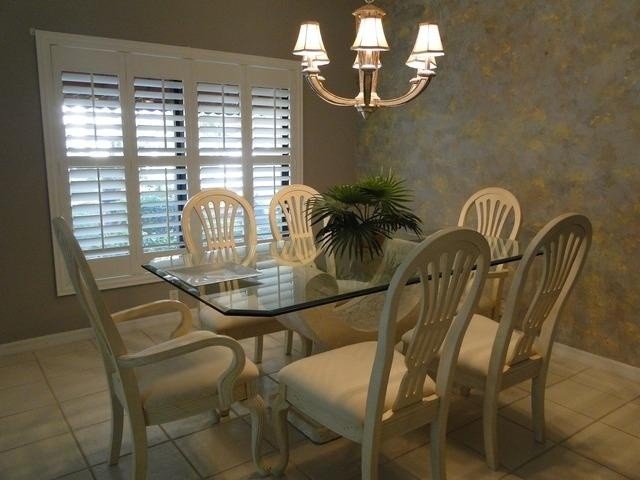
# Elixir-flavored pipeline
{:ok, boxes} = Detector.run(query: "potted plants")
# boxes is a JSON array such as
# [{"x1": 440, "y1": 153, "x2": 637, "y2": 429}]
[{"x1": 302, "y1": 164, "x2": 424, "y2": 261}]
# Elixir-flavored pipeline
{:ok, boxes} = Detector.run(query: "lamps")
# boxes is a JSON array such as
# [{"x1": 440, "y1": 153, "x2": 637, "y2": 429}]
[{"x1": 293, "y1": 0, "x2": 445, "y2": 121}]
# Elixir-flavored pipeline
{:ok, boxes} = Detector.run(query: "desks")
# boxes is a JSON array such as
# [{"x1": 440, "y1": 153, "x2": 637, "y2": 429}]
[{"x1": 141, "y1": 233, "x2": 544, "y2": 446}]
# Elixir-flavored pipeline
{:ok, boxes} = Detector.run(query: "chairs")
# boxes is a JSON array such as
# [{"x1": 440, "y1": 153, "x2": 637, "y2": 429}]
[
  {"x1": 268, "y1": 184, "x2": 331, "y2": 355},
  {"x1": 457, "y1": 188, "x2": 521, "y2": 398},
  {"x1": 181, "y1": 188, "x2": 264, "y2": 363},
  {"x1": 272, "y1": 227, "x2": 491, "y2": 479},
  {"x1": 401, "y1": 213, "x2": 592, "y2": 471},
  {"x1": 53, "y1": 217, "x2": 271, "y2": 480}
]
